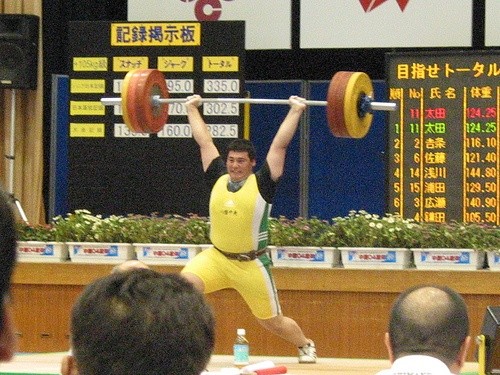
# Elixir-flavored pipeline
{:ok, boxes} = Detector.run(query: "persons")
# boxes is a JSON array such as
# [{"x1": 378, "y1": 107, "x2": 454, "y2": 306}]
[
  {"x1": 177, "y1": 94, "x2": 317, "y2": 363},
  {"x1": 0, "y1": 191, "x2": 17, "y2": 363},
  {"x1": 373, "y1": 284, "x2": 473, "y2": 375},
  {"x1": 61, "y1": 269, "x2": 216, "y2": 375}
]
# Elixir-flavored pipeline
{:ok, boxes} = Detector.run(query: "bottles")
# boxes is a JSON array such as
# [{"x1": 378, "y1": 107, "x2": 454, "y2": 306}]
[{"x1": 232, "y1": 329, "x2": 249, "y2": 368}]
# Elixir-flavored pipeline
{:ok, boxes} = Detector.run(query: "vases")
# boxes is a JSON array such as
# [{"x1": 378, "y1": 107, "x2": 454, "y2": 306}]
[
  {"x1": 266, "y1": 246, "x2": 341, "y2": 268},
  {"x1": 200, "y1": 244, "x2": 215, "y2": 252},
  {"x1": 133, "y1": 242, "x2": 213, "y2": 266},
  {"x1": 337, "y1": 247, "x2": 410, "y2": 269},
  {"x1": 483, "y1": 250, "x2": 500, "y2": 270},
  {"x1": 14, "y1": 240, "x2": 67, "y2": 262},
  {"x1": 64, "y1": 241, "x2": 135, "y2": 264},
  {"x1": 410, "y1": 249, "x2": 485, "y2": 271}
]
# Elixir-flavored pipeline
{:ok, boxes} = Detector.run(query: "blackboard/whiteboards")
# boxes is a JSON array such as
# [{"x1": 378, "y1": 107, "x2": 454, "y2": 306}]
[{"x1": 67, "y1": 18, "x2": 246, "y2": 215}]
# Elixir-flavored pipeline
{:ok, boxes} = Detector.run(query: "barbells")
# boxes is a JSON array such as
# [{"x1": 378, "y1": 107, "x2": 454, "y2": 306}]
[{"x1": 99, "y1": 69, "x2": 398, "y2": 140}]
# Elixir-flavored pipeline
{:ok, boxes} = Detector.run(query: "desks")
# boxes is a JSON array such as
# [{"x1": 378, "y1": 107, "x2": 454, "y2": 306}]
[{"x1": 0, "y1": 350, "x2": 482, "y2": 375}]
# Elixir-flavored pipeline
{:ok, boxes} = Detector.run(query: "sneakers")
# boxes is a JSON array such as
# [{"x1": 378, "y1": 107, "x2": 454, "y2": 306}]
[{"x1": 298, "y1": 339, "x2": 316, "y2": 362}]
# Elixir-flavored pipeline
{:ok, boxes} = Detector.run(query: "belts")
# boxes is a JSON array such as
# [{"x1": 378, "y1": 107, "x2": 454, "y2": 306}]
[{"x1": 214, "y1": 247, "x2": 266, "y2": 262}]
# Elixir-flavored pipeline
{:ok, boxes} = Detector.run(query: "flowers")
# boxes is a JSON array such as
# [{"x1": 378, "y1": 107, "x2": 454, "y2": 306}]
[{"x1": 17, "y1": 210, "x2": 500, "y2": 250}]
